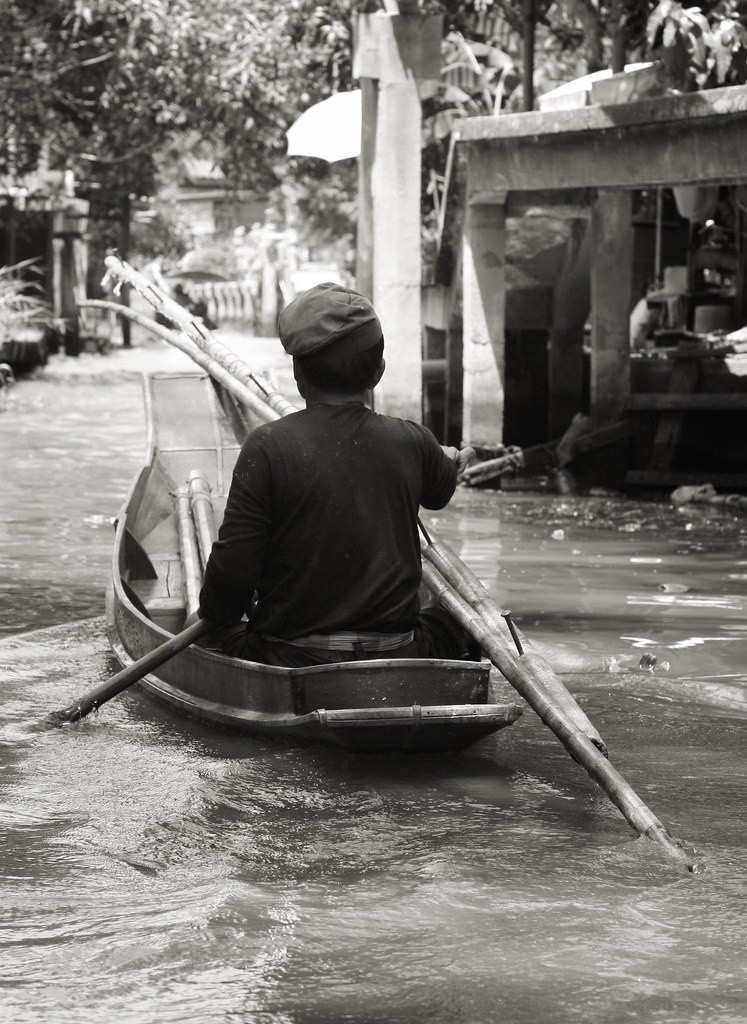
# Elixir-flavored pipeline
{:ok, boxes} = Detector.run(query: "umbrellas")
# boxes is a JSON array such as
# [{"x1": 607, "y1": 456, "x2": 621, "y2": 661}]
[{"x1": 287, "y1": 87, "x2": 362, "y2": 163}]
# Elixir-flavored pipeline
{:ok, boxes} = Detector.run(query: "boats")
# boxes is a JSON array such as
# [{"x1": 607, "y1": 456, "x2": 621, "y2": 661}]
[
  {"x1": 152, "y1": 308, "x2": 219, "y2": 334},
  {"x1": 106, "y1": 369, "x2": 524, "y2": 742}
]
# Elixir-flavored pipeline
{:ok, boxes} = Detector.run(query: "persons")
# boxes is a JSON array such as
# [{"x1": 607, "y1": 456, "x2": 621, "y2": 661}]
[
  {"x1": 187, "y1": 282, "x2": 479, "y2": 665},
  {"x1": 174, "y1": 285, "x2": 192, "y2": 306}
]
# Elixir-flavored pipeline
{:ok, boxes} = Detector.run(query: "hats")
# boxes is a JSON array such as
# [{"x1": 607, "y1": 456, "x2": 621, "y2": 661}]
[{"x1": 277, "y1": 282, "x2": 382, "y2": 357}]
